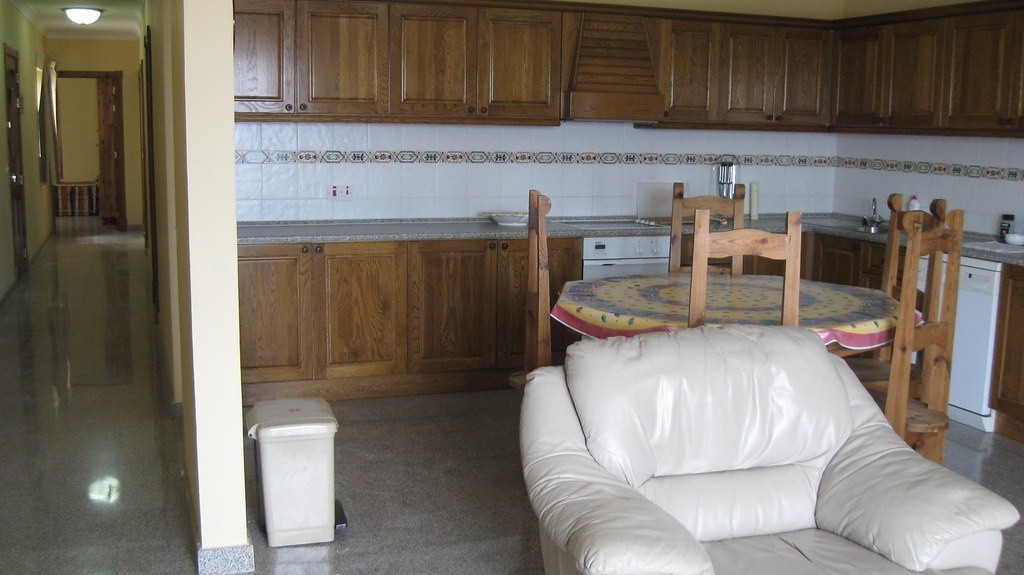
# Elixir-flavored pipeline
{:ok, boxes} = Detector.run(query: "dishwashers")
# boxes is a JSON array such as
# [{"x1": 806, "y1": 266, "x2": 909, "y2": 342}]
[{"x1": 910, "y1": 253, "x2": 1002, "y2": 417}]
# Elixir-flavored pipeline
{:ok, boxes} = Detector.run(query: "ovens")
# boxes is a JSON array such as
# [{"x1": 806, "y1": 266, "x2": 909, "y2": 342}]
[{"x1": 583, "y1": 236, "x2": 672, "y2": 279}]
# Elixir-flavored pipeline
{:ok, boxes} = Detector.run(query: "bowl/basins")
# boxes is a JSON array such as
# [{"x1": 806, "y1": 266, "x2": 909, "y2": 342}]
[
  {"x1": 492, "y1": 214, "x2": 530, "y2": 225},
  {"x1": 1005, "y1": 234, "x2": 1024, "y2": 245}
]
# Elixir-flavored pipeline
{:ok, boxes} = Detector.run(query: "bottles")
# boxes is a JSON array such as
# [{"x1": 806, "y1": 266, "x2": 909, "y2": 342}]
[
  {"x1": 908, "y1": 195, "x2": 920, "y2": 210},
  {"x1": 996, "y1": 214, "x2": 1015, "y2": 243}
]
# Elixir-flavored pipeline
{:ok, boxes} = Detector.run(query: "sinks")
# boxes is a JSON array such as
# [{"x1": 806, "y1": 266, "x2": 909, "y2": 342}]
[
  {"x1": 555, "y1": 219, "x2": 665, "y2": 229},
  {"x1": 804, "y1": 216, "x2": 868, "y2": 227}
]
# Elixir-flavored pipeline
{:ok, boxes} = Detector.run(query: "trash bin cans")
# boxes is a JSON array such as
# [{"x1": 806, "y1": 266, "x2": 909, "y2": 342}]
[{"x1": 245, "y1": 395, "x2": 340, "y2": 548}]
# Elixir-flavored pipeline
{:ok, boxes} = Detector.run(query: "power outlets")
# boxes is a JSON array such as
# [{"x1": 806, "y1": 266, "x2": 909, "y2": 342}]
[{"x1": 328, "y1": 184, "x2": 353, "y2": 200}]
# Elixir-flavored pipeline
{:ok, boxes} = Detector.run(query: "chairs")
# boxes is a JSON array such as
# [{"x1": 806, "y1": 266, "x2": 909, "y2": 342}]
[{"x1": 528, "y1": 180, "x2": 965, "y2": 459}]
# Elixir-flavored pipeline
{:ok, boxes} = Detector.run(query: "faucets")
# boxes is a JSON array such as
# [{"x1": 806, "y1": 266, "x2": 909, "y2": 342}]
[{"x1": 860, "y1": 196, "x2": 887, "y2": 224}]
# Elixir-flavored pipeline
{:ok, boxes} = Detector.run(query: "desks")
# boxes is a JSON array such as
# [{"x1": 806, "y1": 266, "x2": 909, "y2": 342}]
[{"x1": 546, "y1": 269, "x2": 924, "y2": 357}]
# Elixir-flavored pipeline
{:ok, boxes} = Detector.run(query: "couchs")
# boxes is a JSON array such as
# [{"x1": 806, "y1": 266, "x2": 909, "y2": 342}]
[{"x1": 520, "y1": 322, "x2": 1017, "y2": 575}]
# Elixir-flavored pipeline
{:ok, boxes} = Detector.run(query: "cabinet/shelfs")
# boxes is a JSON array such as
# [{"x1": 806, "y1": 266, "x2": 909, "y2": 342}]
[
  {"x1": 830, "y1": 18, "x2": 885, "y2": 134},
  {"x1": 712, "y1": 15, "x2": 838, "y2": 129},
  {"x1": 988, "y1": 267, "x2": 1024, "y2": 439},
  {"x1": 234, "y1": 1, "x2": 389, "y2": 121},
  {"x1": 642, "y1": 16, "x2": 719, "y2": 129},
  {"x1": 877, "y1": 0, "x2": 1024, "y2": 136},
  {"x1": 236, "y1": 247, "x2": 415, "y2": 405},
  {"x1": 807, "y1": 236, "x2": 902, "y2": 284},
  {"x1": 387, "y1": 0, "x2": 564, "y2": 124},
  {"x1": 411, "y1": 238, "x2": 582, "y2": 397}
]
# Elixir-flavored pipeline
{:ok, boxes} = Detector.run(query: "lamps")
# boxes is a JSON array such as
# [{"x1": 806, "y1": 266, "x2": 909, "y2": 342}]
[{"x1": 62, "y1": 8, "x2": 103, "y2": 25}]
